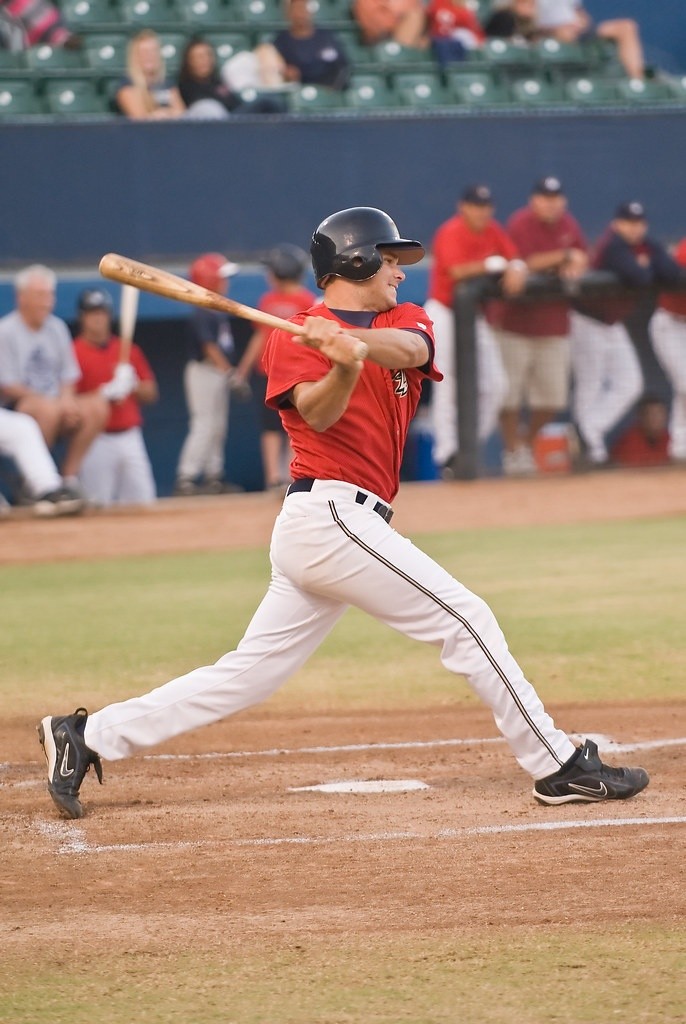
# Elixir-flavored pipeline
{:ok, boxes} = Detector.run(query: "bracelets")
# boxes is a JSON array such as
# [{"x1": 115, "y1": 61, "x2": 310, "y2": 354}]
[{"x1": 559, "y1": 247, "x2": 574, "y2": 263}]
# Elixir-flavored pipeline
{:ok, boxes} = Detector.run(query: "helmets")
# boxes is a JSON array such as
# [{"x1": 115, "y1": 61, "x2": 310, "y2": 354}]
[{"x1": 309, "y1": 207, "x2": 425, "y2": 289}]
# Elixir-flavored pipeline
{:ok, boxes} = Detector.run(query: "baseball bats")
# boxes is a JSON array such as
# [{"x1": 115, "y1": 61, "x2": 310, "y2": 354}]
[
  {"x1": 115, "y1": 283, "x2": 141, "y2": 406},
  {"x1": 98, "y1": 252, "x2": 368, "y2": 360}
]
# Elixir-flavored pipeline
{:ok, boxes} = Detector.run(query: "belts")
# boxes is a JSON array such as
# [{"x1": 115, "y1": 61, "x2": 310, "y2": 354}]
[{"x1": 287, "y1": 476, "x2": 394, "y2": 524}]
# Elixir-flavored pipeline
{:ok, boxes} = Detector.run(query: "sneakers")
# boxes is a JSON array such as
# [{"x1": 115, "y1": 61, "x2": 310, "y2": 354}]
[
  {"x1": 34, "y1": 490, "x2": 81, "y2": 516},
  {"x1": 36, "y1": 707, "x2": 102, "y2": 818},
  {"x1": 532, "y1": 738, "x2": 650, "y2": 805}
]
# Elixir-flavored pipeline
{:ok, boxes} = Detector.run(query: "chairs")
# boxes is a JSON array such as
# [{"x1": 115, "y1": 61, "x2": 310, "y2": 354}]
[{"x1": 0, "y1": 0, "x2": 686, "y2": 121}]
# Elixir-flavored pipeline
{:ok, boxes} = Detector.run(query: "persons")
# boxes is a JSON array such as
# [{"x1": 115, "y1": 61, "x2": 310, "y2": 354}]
[
  {"x1": 0, "y1": 265, "x2": 161, "y2": 517},
  {"x1": 0, "y1": 0, "x2": 81, "y2": 50},
  {"x1": 489, "y1": 175, "x2": 589, "y2": 474},
  {"x1": 420, "y1": 185, "x2": 529, "y2": 482},
  {"x1": 37, "y1": 207, "x2": 654, "y2": 819},
  {"x1": 172, "y1": 252, "x2": 254, "y2": 497},
  {"x1": 102, "y1": 0, "x2": 648, "y2": 122},
  {"x1": 569, "y1": 198, "x2": 686, "y2": 469},
  {"x1": 234, "y1": 242, "x2": 318, "y2": 488}
]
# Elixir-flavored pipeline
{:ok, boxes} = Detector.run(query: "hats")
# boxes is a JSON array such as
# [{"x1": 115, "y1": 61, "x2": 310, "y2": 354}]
[
  {"x1": 80, "y1": 289, "x2": 111, "y2": 309},
  {"x1": 271, "y1": 244, "x2": 305, "y2": 278},
  {"x1": 536, "y1": 177, "x2": 563, "y2": 196},
  {"x1": 618, "y1": 201, "x2": 646, "y2": 220},
  {"x1": 191, "y1": 254, "x2": 239, "y2": 289},
  {"x1": 464, "y1": 186, "x2": 494, "y2": 205}
]
